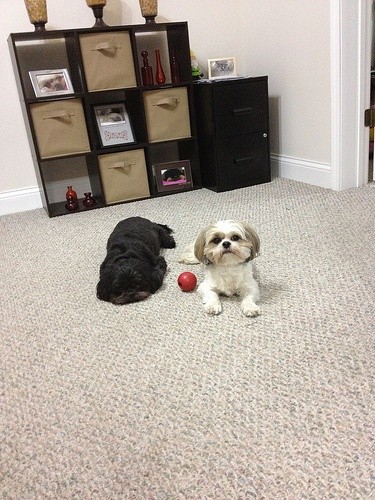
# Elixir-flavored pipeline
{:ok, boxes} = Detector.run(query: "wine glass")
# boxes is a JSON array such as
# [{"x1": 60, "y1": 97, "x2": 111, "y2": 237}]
[
  {"x1": 139, "y1": 0, "x2": 158, "y2": 24},
  {"x1": 24, "y1": 0, "x2": 48, "y2": 32},
  {"x1": 85, "y1": 0, "x2": 110, "y2": 28}
]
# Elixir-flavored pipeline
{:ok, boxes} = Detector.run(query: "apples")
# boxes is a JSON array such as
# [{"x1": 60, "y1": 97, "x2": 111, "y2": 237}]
[{"x1": 178, "y1": 272, "x2": 196, "y2": 291}]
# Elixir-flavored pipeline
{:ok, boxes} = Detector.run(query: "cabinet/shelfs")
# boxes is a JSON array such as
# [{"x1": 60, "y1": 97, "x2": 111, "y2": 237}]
[
  {"x1": 193, "y1": 74, "x2": 271, "y2": 194},
  {"x1": 7, "y1": 21, "x2": 202, "y2": 218}
]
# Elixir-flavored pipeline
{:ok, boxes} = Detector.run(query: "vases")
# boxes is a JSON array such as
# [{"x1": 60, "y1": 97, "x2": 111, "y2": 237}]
[
  {"x1": 85, "y1": 0, "x2": 110, "y2": 27},
  {"x1": 140, "y1": 50, "x2": 179, "y2": 87},
  {"x1": 65, "y1": 186, "x2": 95, "y2": 212},
  {"x1": 24, "y1": 0, "x2": 48, "y2": 32},
  {"x1": 139, "y1": 0, "x2": 158, "y2": 24}
]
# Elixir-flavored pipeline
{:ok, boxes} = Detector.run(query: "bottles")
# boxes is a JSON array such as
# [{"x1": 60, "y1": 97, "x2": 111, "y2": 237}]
[
  {"x1": 170, "y1": 49, "x2": 180, "y2": 83},
  {"x1": 155, "y1": 49, "x2": 165, "y2": 85},
  {"x1": 65, "y1": 186, "x2": 79, "y2": 207},
  {"x1": 191, "y1": 56, "x2": 203, "y2": 81},
  {"x1": 65, "y1": 194, "x2": 77, "y2": 212},
  {"x1": 141, "y1": 51, "x2": 154, "y2": 86},
  {"x1": 82, "y1": 192, "x2": 95, "y2": 207}
]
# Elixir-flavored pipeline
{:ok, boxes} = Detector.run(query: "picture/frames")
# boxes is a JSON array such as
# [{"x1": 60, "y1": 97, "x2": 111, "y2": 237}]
[
  {"x1": 94, "y1": 102, "x2": 138, "y2": 148},
  {"x1": 29, "y1": 68, "x2": 75, "y2": 98},
  {"x1": 154, "y1": 159, "x2": 193, "y2": 193},
  {"x1": 208, "y1": 57, "x2": 238, "y2": 80}
]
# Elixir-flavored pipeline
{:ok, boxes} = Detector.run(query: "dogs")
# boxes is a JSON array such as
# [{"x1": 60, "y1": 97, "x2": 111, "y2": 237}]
[
  {"x1": 96, "y1": 216, "x2": 175, "y2": 305},
  {"x1": 178, "y1": 221, "x2": 260, "y2": 318}
]
